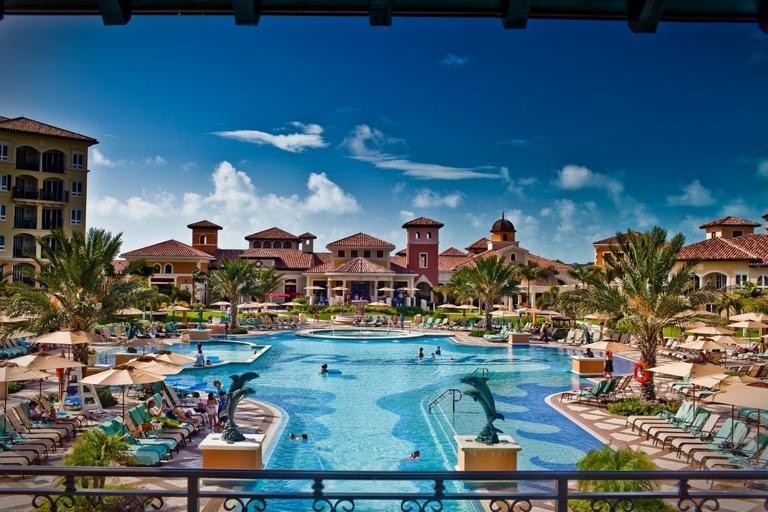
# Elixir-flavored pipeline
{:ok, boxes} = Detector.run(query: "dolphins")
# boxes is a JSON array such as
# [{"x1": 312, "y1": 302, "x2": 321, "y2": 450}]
[
  {"x1": 462, "y1": 390, "x2": 505, "y2": 434},
  {"x1": 222, "y1": 371, "x2": 260, "y2": 405},
  {"x1": 459, "y1": 376, "x2": 507, "y2": 423},
  {"x1": 225, "y1": 386, "x2": 256, "y2": 426}
]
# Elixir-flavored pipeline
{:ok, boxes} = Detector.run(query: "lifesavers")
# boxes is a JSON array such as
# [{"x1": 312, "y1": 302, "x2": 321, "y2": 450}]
[
  {"x1": 633, "y1": 363, "x2": 650, "y2": 382},
  {"x1": 326, "y1": 368, "x2": 341, "y2": 375}
]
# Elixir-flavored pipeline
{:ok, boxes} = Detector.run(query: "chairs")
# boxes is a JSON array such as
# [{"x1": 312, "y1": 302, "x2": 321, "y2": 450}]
[
  {"x1": 556, "y1": 329, "x2": 768, "y2": 490},
  {"x1": 358, "y1": 310, "x2": 560, "y2": 346},
  {"x1": 219, "y1": 314, "x2": 311, "y2": 331},
  {"x1": 0, "y1": 321, "x2": 210, "y2": 481}
]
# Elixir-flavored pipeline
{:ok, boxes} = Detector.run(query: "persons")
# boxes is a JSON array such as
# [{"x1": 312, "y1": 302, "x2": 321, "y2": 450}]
[
  {"x1": 147, "y1": 322, "x2": 163, "y2": 336},
  {"x1": 585, "y1": 348, "x2": 614, "y2": 378},
  {"x1": 407, "y1": 450, "x2": 422, "y2": 460},
  {"x1": 224, "y1": 322, "x2": 229, "y2": 336},
  {"x1": 28, "y1": 401, "x2": 80, "y2": 423},
  {"x1": 355, "y1": 313, "x2": 404, "y2": 330},
  {"x1": 148, "y1": 381, "x2": 229, "y2": 431}
]
